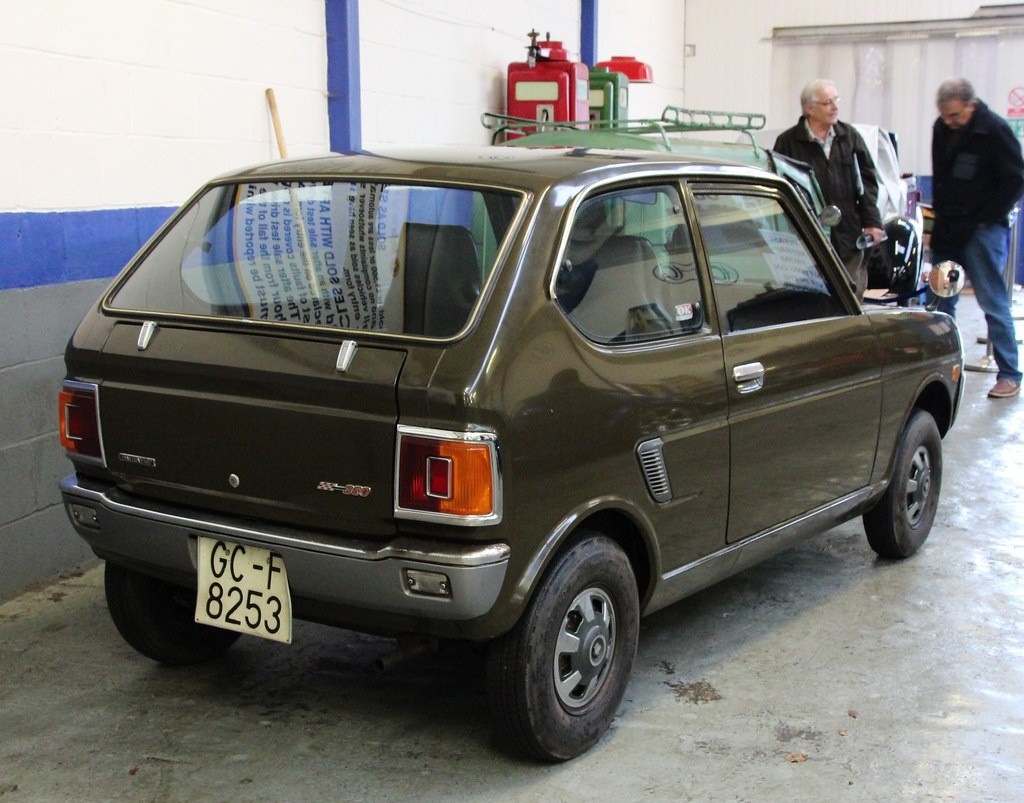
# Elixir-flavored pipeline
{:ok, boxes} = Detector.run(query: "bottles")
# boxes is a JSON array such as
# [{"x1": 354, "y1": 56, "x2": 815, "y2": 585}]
[{"x1": 1008, "y1": 207, "x2": 1018, "y2": 227}]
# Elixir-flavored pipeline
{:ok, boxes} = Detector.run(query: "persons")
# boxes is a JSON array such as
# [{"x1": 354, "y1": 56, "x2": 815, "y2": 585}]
[
  {"x1": 773, "y1": 79, "x2": 882, "y2": 305},
  {"x1": 947, "y1": 263, "x2": 959, "y2": 295},
  {"x1": 929, "y1": 77, "x2": 1024, "y2": 398}
]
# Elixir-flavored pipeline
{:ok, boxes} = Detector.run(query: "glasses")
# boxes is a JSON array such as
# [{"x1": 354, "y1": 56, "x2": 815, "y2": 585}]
[
  {"x1": 940, "y1": 106, "x2": 967, "y2": 120},
  {"x1": 816, "y1": 95, "x2": 840, "y2": 107}
]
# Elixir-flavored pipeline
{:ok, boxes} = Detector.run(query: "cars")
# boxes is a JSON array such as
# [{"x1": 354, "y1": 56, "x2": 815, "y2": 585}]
[{"x1": 53, "y1": 104, "x2": 967, "y2": 766}]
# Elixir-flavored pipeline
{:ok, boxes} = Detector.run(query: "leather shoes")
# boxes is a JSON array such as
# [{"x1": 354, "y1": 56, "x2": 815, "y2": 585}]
[{"x1": 988, "y1": 378, "x2": 1020, "y2": 398}]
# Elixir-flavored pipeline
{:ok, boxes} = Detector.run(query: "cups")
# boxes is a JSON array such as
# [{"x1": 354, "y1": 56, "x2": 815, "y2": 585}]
[{"x1": 901, "y1": 173, "x2": 917, "y2": 192}]
